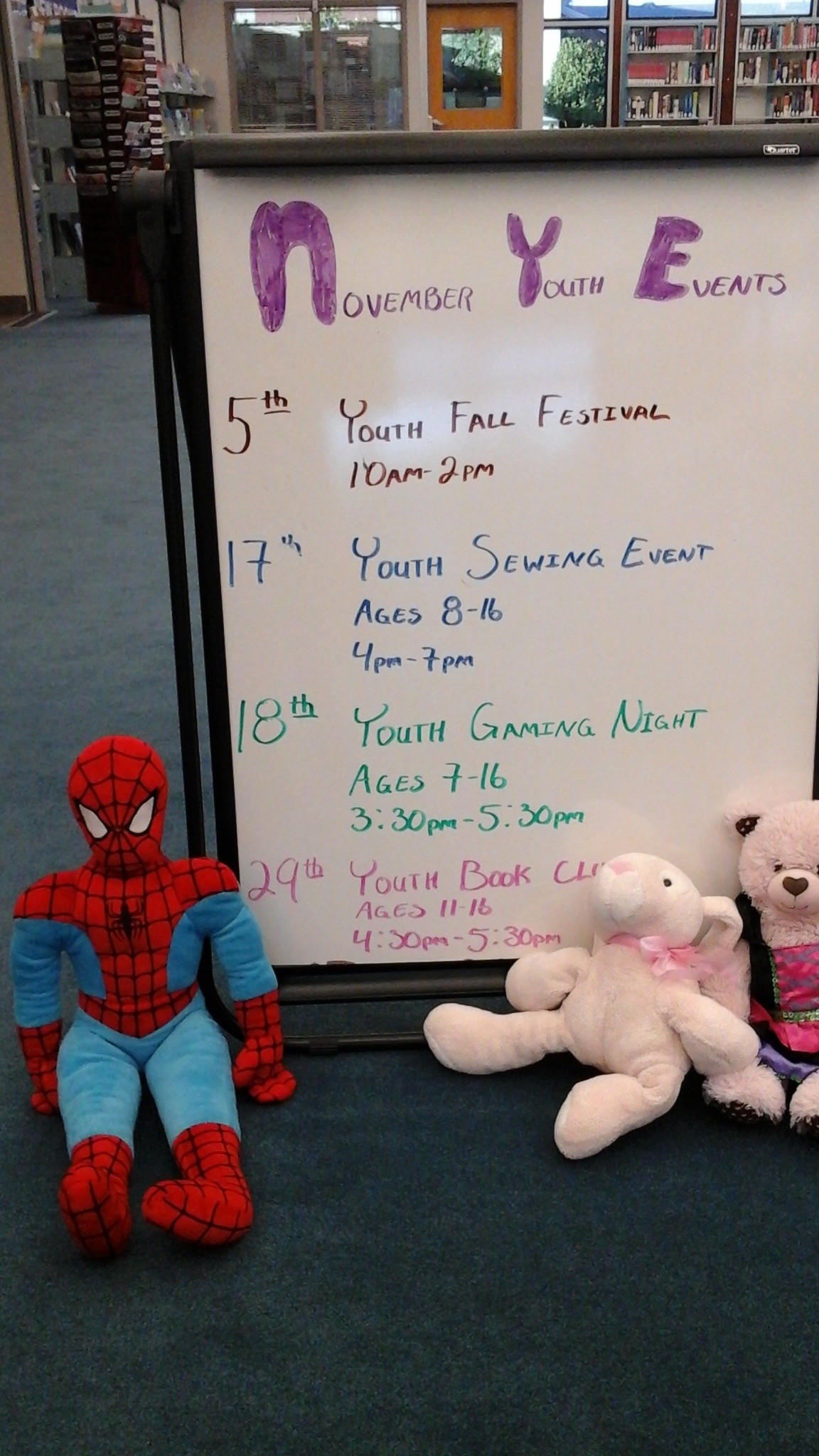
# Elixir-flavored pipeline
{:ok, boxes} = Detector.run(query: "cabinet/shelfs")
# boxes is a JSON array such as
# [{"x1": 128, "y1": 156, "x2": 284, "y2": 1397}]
[
  {"x1": 624, "y1": 23, "x2": 719, "y2": 127},
  {"x1": 738, "y1": 21, "x2": 819, "y2": 124}
]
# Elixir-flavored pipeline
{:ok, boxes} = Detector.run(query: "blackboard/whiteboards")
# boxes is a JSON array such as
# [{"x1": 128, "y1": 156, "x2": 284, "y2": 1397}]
[{"x1": 177, "y1": 123, "x2": 819, "y2": 1005}]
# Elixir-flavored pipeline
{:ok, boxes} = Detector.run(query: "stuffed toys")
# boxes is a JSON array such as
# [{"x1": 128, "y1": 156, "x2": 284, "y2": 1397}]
[
  {"x1": 11, "y1": 734, "x2": 298, "y2": 1261},
  {"x1": 424, "y1": 799, "x2": 819, "y2": 1157}
]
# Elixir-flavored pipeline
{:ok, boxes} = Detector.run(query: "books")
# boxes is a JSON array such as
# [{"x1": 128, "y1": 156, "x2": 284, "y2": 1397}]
[{"x1": 627, "y1": 15, "x2": 819, "y2": 121}]
